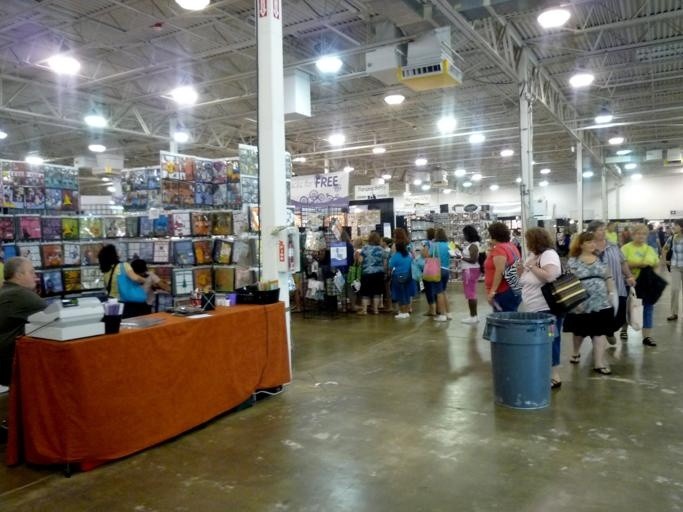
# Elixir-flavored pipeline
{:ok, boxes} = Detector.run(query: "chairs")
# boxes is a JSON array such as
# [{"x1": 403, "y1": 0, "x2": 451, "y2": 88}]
[{"x1": 0, "y1": 314, "x2": 27, "y2": 430}]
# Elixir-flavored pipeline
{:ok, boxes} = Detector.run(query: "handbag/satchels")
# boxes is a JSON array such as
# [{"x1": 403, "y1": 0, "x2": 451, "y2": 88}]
[
  {"x1": 398, "y1": 272, "x2": 410, "y2": 283},
  {"x1": 626, "y1": 286, "x2": 644, "y2": 330},
  {"x1": 117, "y1": 263, "x2": 147, "y2": 302},
  {"x1": 536, "y1": 247, "x2": 591, "y2": 316},
  {"x1": 635, "y1": 265, "x2": 666, "y2": 306},
  {"x1": 494, "y1": 244, "x2": 524, "y2": 296},
  {"x1": 665, "y1": 234, "x2": 674, "y2": 271},
  {"x1": 422, "y1": 243, "x2": 441, "y2": 282},
  {"x1": 347, "y1": 260, "x2": 363, "y2": 294}
]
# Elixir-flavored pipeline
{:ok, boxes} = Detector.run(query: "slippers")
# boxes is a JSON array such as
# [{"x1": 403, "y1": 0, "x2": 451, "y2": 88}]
[
  {"x1": 551, "y1": 378, "x2": 561, "y2": 389},
  {"x1": 620, "y1": 330, "x2": 627, "y2": 340},
  {"x1": 643, "y1": 336, "x2": 657, "y2": 346},
  {"x1": 593, "y1": 367, "x2": 612, "y2": 375},
  {"x1": 570, "y1": 354, "x2": 580, "y2": 363}
]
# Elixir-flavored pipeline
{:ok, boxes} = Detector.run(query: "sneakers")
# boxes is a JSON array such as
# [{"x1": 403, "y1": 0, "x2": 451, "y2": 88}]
[
  {"x1": 461, "y1": 314, "x2": 479, "y2": 324},
  {"x1": 356, "y1": 306, "x2": 413, "y2": 318},
  {"x1": 667, "y1": 315, "x2": 678, "y2": 321},
  {"x1": 422, "y1": 311, "x2": 452, "y2": 321}
]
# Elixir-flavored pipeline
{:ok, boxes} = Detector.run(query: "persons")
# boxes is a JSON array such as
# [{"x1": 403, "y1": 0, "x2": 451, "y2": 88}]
[
  {"x1": 518, "y1": 228, "x2": 563, "y2": 388},
  {"x1": 556, "y1": 221, "x2": 681, "y2": 374},
  {"x1": 421, "y1": 227, "x2": 452, "y2": 322},
  {"x1": 98, "y1": 244, "x2": 151, "y2": 318},
  {"x1": 355, "y1": 228, "x2": 416, "y2": 319},
  {"x1": 484, "y1": 222, "x2": 522, "y2": 312},
  {"x1": 131, "y1": 259, "x2": 172, "y2": 314},
  {"x1": 456, "y1": 225, "x2": 482, "y2": 323},
  {"x1": 0, "y1": 256, "x2": 49, "y2": 386}
]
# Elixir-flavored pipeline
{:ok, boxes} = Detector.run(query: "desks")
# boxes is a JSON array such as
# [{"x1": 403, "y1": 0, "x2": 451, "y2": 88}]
[{"x1": 6, "y1": 301, "x2": 291, "y2": 478}]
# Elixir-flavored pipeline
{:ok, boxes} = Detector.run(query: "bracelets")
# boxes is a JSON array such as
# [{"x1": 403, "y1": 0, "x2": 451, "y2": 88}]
[
  {"x1": 461, "y1": 256, "x2": 463, "y2": 259},
  {"x1": 491, "y1": 292, "x2": 496, "y2": 294},
  {"x1": 529, "y1": 265, "x2": 532, "y2": 269}
]
